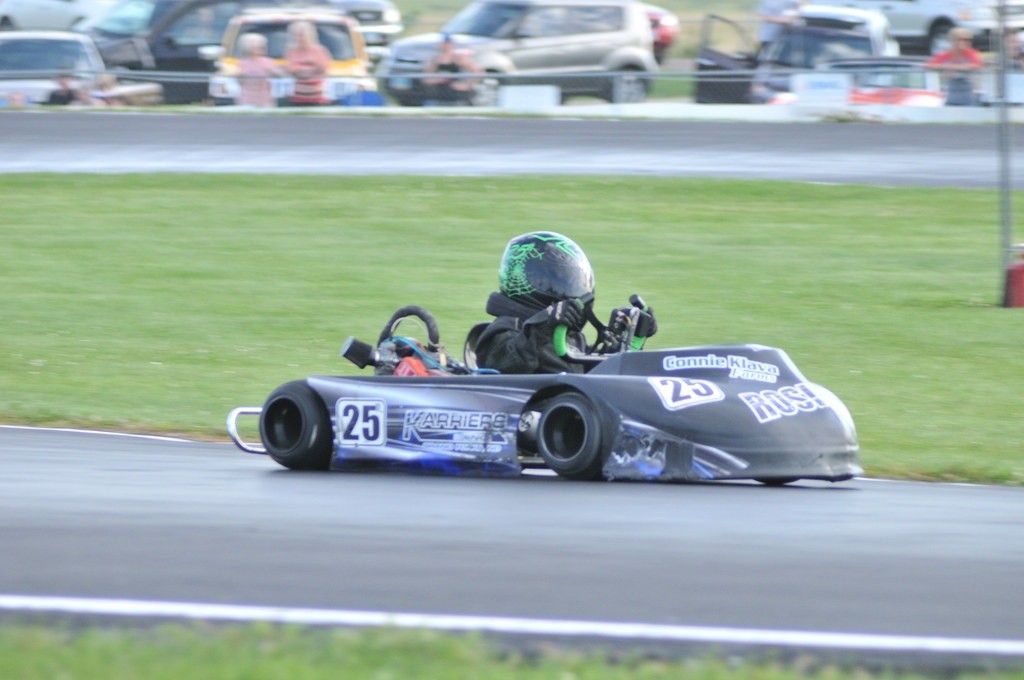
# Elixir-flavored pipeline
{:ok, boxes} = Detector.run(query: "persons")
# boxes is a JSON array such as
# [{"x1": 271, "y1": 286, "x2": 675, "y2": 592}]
[
  {"x1": 925, "y1": 25, "x2": 984, "y2": 106},
  {"x1": 231, "y1": 19, "x2": 332, "y2": 106},
  {"x1": 419, "y1": 31, "x2": 482, "y2": 106},
  {"x1": 475, "y1": 231, "x2": 657, "y2": 373},
  {"x1": 44, "y1": 64, "x2": 133, "y2": 107}
]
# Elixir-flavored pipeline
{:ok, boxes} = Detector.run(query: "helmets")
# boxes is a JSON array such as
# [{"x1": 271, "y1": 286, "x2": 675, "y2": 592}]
[{"x1": 498, "y1": 231, "x2": 596, "y2": 339}]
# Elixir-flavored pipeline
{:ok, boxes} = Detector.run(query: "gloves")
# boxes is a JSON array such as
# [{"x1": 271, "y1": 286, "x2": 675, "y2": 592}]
[
  {"x1": 543, "y1": 298, "x2": 582, "y2": 329},
  {"x1": 634, "y1": 307, "x2": 658, "y2": 338}
]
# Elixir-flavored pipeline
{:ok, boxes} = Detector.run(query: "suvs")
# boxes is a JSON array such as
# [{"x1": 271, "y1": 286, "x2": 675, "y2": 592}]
[{"x1": 385, "y1": 1, "x2": 664, "y2": 106}]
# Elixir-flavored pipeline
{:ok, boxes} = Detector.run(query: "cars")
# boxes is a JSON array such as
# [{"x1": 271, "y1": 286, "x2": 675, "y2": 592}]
[
  {"x1": 645, "y1": 0, "x2": 1024, "y2": 109},
  {"x1": 0, "y1": 0, "x2": 406, "y2": 108}
]
